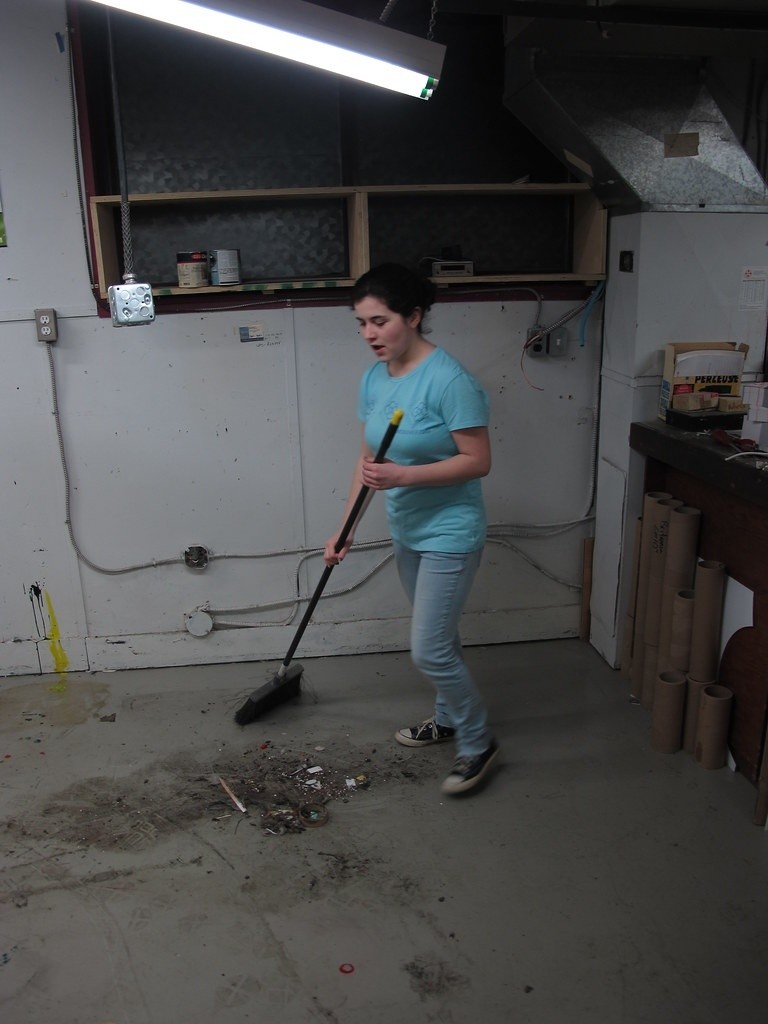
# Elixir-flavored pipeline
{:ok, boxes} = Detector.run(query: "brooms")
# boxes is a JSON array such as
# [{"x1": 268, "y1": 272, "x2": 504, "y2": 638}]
[{"x1": 232, "y1": 408, "x2": 405, "y2": 727}]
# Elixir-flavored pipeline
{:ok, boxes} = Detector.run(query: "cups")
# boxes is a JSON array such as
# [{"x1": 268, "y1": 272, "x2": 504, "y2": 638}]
[{"x1": 176, "y1": 248, "x2": 243, "y2": 289}]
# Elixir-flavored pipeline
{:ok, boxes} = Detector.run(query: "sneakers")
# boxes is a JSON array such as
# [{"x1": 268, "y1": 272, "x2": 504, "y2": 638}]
[
  {"x1": 394, "y1": 708, "x2": 461, "y2": 747},
  {"x1": 440, "y1": 739, "x2": 503, "y2": 793}
]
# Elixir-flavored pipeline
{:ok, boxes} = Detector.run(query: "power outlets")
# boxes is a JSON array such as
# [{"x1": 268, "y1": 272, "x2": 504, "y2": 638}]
[
  {"x1": 526, "y1": 327, "x2": 547, "y2": 357},
  {"x1": 34, "y1": 308, "x2": 58, "y2": 343}
]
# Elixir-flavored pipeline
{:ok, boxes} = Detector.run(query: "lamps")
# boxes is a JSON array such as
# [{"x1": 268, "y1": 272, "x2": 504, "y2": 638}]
[{"x1": 91, "y1": 0, "x2": 449, "y2": 102}]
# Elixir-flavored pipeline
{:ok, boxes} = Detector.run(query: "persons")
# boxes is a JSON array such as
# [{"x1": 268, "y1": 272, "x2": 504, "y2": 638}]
[{"x1": 324, "y1": 264, "x2": 500, "y2": 793}]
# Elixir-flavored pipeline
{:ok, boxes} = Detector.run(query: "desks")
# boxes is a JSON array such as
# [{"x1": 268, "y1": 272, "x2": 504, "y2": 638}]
[{"x1": 636, "y1": 417, "x2": 768, "y2": 787}]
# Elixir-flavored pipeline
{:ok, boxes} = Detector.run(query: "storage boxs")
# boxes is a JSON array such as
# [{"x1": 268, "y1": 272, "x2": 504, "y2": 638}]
[{"x1": 656, "y1": 341, "x2": 768, "y2": 452}]
